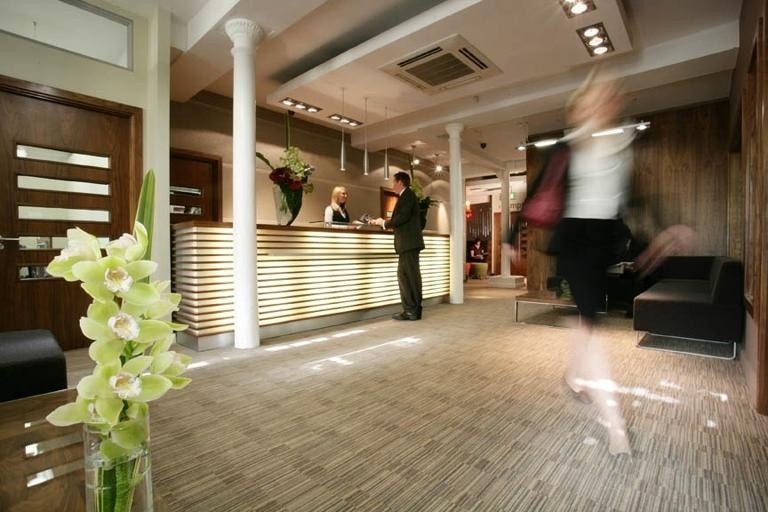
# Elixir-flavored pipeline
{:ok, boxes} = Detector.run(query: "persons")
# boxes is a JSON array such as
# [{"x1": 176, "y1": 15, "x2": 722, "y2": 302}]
[
  {"x1": 324, "y1": 185, "x2": 349, "y2": 225},
  {"x1": 368, "y1": 172, "x2": 426, "y2": 319},
  {"x1": 469, "y1": 238, "x2": 489, "y2": 263},
  {"x1": 517, "y1": 81, "x2": 698, "y2": 477}
]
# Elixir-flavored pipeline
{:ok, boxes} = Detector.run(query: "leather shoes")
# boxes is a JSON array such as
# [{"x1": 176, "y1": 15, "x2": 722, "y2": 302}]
[{"x1": 392, "y1": 310, "x2": 417, "y2": 320}]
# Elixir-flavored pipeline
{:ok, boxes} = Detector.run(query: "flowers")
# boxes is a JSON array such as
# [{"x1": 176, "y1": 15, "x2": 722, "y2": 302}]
[
  {"x1": 256, "y1": 101, "x2": 314, "y2": 226},
  {"x1": 407, "y1": 153, "x2": 442, "y2": 215},
  {"x1": 39, "y1": 166, "x2": 200, "y2": 512}
]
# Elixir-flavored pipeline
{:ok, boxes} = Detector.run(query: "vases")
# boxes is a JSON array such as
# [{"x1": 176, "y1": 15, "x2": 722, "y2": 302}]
[{"x1": 78, "y1": 405, "x2": 157, "y2": 512}]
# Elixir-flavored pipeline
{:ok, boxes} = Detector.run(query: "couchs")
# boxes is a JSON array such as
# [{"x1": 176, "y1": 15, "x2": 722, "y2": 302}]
[
  {"x1": 600, "y1": 255, "x2": 745, "y2": 361},
  {"x1": 1, "y1": 329, "x2": 67, "y2": 403}
]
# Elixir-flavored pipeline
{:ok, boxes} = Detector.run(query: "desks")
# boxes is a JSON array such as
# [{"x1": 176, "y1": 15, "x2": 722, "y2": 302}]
[{"x1": 0, "y1": 386, "x2": 150, "y2": 512}]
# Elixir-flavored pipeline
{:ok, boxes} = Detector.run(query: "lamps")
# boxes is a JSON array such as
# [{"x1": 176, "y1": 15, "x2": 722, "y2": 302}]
[
  {"x1": 381, "y1": 106, "x2": 391, "y2": 180},
  {"x1": 362, "y1": 95, "x2": 370, "y2": 175},
  {"x1": 338, "y1": 86, "x2": 347, "y2": 171}
]
